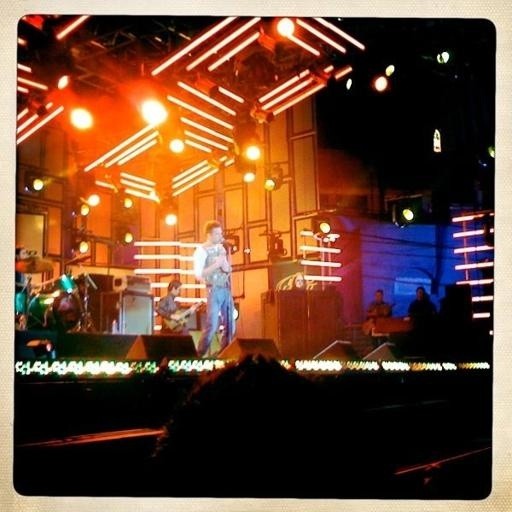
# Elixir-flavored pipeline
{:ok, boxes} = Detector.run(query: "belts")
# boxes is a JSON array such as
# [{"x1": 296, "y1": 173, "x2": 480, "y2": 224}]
[{"x1": 206, "y1": 284, "x2": 231, "y2": 288}]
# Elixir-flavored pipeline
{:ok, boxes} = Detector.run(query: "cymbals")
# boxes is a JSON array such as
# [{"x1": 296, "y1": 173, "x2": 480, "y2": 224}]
[
  {"x1": 64, "y1": 254, "x2": 94, "y2": 267},
  {"x1": 15, "y1": 257, "x2": 55, "y2": 274}
]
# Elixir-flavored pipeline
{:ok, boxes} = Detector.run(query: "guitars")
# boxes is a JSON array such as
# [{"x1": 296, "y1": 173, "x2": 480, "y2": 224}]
[
  {"x1": 362, "y1": 302, "x2": 395, "y2": 336},
  {"x1": 164, "y1": 300, "x2": 204, "y2": 333}
]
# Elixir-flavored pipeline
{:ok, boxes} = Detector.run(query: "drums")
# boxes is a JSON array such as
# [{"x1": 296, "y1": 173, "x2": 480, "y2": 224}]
[
  {"x1": 53, "y1": 274, "x2": 79, "y2": 297},
  {"x1": 26, "y1": 291, "x2": 80, "y2": 332}
]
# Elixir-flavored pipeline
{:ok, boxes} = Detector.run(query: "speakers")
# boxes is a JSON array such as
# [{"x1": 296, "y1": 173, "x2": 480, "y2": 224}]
[
  {"x1": 273, "y1": 261, "x2": 321, "y2": 289},
  {"x1": 126, "y1": 334, "x2": 199, "y2": 361},
  {"x1": 446, "y1": 284, "x2": 472, "y2": 322},
  {"x1": 101, "y1": 291, "x2": 153, "y2": 336},
  {"x1": 362, "y1": 343, "x2": 403, "y2": 360},
  {"x1": 88, "y1": 274, "x2": 114, "y2": 332},
  {"x1": 217, "y1": 337, "x2": 282, "y2": 359},
  {"x1": 312, "y1": 340, "x2": 360, "y2": 361}
]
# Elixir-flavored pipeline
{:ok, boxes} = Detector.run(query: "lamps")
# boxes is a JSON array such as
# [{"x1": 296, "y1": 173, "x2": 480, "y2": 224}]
[{"x1": 24, "y1": 15, "x2": 496, "y2": 259}]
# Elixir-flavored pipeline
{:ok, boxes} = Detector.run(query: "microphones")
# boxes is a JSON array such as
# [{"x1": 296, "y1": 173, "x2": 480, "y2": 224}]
[{"x1": 220, "y1": 237, "x2": 232, "y2": 247}]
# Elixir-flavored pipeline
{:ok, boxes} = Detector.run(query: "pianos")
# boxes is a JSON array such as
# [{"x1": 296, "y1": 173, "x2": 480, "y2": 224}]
[{"x1": 373, "y1": 317, "x2": 415, "y2": 333}]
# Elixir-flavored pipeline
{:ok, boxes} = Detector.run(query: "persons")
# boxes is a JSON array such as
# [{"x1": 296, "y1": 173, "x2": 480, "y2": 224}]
[
  {"x1": 193, "y1": 220, "x2": 236, "y2": 359},
  {"x1": 142, "y1": 352, "x2": 361, "y2": 496},
  {"x1": 408, "y1": 287, "x2": 437, "y2": 317},
  {"x1": 155, "y1": 279, "x2": 196, "y2": 335},
  {"x1": 367, "y1": 290, "x2": 392, "y2": 320}
]
